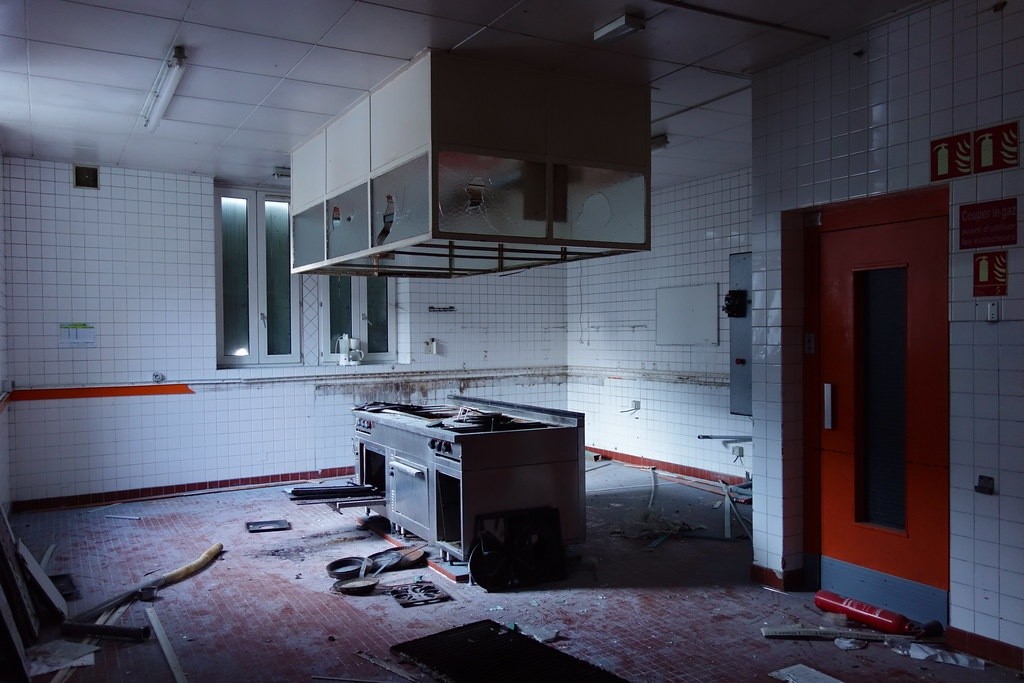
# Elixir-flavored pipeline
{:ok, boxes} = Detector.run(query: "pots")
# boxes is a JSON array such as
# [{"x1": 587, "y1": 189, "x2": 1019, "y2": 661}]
[
  {"x1": 327, "y1": 556, "x2": 380, "y2": 596},
  {"x1": 368, "y1": 545, "x2": 425, "y2": 572}
]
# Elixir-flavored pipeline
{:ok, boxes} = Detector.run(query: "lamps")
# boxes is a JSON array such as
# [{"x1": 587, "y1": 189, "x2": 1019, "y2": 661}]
[{"x1": 144, "y1": 45, "x2": 189, "y2": 134}]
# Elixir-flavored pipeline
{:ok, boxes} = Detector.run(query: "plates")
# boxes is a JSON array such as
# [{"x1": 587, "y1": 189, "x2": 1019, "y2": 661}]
[{"x1": 453, "y1": 412, "x2": 515, "y2": 424}]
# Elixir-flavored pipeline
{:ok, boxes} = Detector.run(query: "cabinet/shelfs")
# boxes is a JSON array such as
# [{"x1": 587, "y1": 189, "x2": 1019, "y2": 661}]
[{"x1": 283, "y1": 436, "x2": 586, "y2": 565}]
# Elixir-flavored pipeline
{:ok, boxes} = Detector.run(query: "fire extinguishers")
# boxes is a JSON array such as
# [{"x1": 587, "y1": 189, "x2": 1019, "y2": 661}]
[{"x1": 814, "y1": 589, "x2": 942, "y2": 641}]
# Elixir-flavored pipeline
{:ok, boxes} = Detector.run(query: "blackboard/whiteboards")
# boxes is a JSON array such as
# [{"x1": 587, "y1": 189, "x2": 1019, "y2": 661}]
[{"x1": 656, "y1": 283, "x2": 719, "y2": 345}]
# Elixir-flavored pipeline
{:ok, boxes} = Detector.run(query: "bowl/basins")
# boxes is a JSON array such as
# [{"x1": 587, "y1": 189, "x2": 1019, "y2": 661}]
[{"x1": 136, "y1": 585, "x2": 158, "y2": 601}]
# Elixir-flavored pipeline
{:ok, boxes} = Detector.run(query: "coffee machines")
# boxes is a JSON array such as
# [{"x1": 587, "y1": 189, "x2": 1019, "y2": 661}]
[{"x1": 338, "y1": 336, "x2": 364, "y2": 366}]
